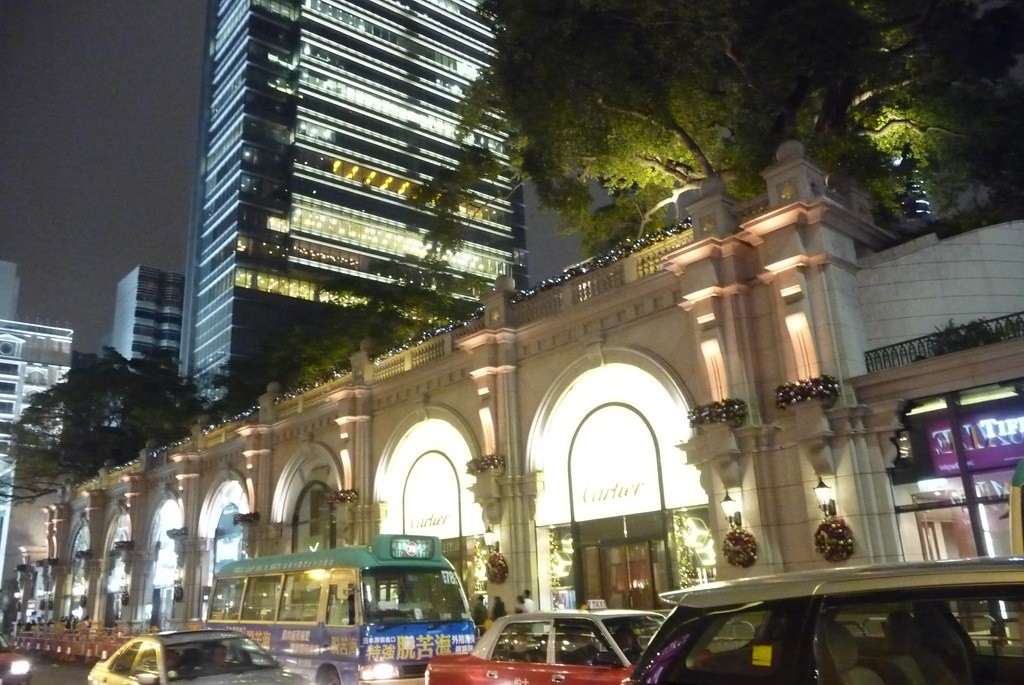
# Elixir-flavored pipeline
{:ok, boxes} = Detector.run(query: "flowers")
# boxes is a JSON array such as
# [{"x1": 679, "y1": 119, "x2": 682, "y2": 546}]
[
  {"x1": 776, "y1": 374, "x2": 841, "y2": 410},
  {"x1": 16, "y1": 602, "x2": 21, "y2": 611},
  {"x1": 39, "y1": 600, "x2": 45, "y2": 610},
  {"x1": 466, "y1": 454, "x2": 504, "y2": 474},
  {"x1": 722, "y1": 530, "x2": 757, "y2": 568},
  {"x1": 233, "y1": 510, "x2": 259, "y2": 527},
  {"x1": 326, "y1": 489, "x2": 358, "y2": 503},
  {"x1": 173, "y1": 588, "x2": 183, "y2": 602},
  {"x1": 121, "y1": 593, "x2": 129, "y2": 606},
  {"x1": 686, "y1": 398, "x2": 746, "y2": 426},
  {"x1": 115, "y1": 541, "x2": 132, "y2": 550},
  {"x1": 816, "y1": 520, "x2": 851, "y2": 559},
  {"x1": 74, "y1": 550, "x2": 93, "y2": 560},
  {"x1": 14, "y1": 558, "x2": 58, "y2": 571},
  {"x1": 166, "y1": 528, "x2": 187, "y2": 538},
  {"x1": 486, "y1": 552, "x2": 507, "y2": 585},
  {"x1": 79, "y1": 595, "x2": 86, "y2": 606}
]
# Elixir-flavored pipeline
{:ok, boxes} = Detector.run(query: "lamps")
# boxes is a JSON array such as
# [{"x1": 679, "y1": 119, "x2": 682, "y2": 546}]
[
  {"x1": 813, "y1": 477, "x2": 836, "y2": 518},
  {"x1": 484, "y1": 526, "x2": 499, "y2": 553},
  {"x1": 719, "y1": 491, "x2": 741, "y2": 529}
]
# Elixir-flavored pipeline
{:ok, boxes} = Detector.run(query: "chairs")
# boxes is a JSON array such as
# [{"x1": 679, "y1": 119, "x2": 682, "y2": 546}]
[
  {"x1": 819, "y1": 621, "x2": 883, "y2": 685},
  {"x1": 878, "y1": 608, "x2": 946, "y2": 685}
]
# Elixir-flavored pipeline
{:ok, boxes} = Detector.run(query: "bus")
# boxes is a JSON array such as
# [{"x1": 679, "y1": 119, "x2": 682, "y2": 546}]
[{"x1": 200, "y1": 535, "x2": 483, "y2": 685}]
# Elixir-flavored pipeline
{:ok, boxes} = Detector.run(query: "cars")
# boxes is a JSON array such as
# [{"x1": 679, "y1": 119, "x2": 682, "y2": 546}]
[
  {"x1": 86, "y1": 628, "x2": 315, "y2": 685},
  {"x1": 420, "y1": 609, "x2": 711, "y2": 685},
  {"x1": 0, "y1": 631, "x2": 33, "y2": 685}
]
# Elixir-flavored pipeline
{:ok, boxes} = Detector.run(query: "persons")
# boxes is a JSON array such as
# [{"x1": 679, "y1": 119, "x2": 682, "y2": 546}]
[
  {"x1": 615, "y1": 627, "x2": 632, "y2": 652},
  {"x1": 17, "y1": 617, "x2": 43, "y2": 631},
  {"x1": 490, "y1": 596, "x2": 505, "y2": 622},
  {"x1": 471, "y1": 595, "x2": 487, "y2": 636},
  {"x1": 210, "y1": 645, "x2": 227, "y2": 666},
  {"x1": 165, "y1": 648, "x2": 178, "y2": 668},
  {"x1": 61, "y1": 614, "x2": 91, "y2": 629},
  {"x1": 514, "y1": 590, "x2": 535, "y2": 633}
]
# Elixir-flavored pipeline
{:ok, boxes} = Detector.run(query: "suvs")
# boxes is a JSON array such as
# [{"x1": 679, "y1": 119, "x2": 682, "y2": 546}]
[{"x1": 617, "y1": 554, "x2": 1024, "y2": 685}]
